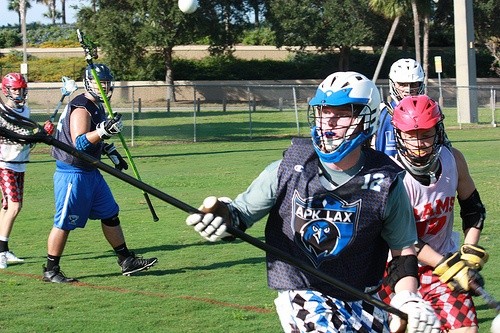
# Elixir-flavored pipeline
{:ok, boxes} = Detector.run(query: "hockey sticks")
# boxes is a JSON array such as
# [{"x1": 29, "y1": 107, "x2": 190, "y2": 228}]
[
  {"x1": 469, "y1": 281, "x2": 500, "y2": 333},
  {"x1": 371, "y1": 17, "x2": 401, "y2": 83},
  {"x1": 76, "y1": 29, "x2": 160, "y2": 222},
  {"x1": 50, "y1": 75, "x2": 78, "y2": 122},
  {"x1": 0, "y1": 107, "x2": 409, "y2": 322}
]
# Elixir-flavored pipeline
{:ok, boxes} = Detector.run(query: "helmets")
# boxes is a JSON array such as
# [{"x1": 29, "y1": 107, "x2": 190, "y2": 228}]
[
  {"x1": 82, "y1": 63, "x2": 115, "y2": 103},
  {"x1": 307, "y1": 71, "x2": 380, "y2": 163},
  {"x1": 390, "y1": 95, "x2": 446, "y2": 175},
  {"x1": 1, "y1": 73, "x2": 28, "y2": 103},
  {"x1": 388, "y1": 58, "x2": 425, "y2": 103}
]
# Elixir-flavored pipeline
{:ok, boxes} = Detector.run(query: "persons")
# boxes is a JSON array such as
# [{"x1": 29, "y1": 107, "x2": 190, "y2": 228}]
[
  {"x1": 42, "y1": 64, "x2": 158, "y2": 283},
  {"x1": 0, "y1": 73, "x2": 55, "y2": 269},
  {"x1": 378, "y1": 95, "x2": 490, "y2": 333},
  {"x1": 185, "y1": 72, "x2": 441, "y2": 333},
  {"x1": 370, "y1": 58, "x2": 451, "y2": 158}
]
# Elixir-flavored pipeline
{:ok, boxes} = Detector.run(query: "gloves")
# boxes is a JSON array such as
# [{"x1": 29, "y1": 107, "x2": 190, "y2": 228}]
[
  {"x1": 43, "y1": 120, "x2": 55, "y2": 135},
  {"x1": 105, "y1": 142, "x2": 128, "y2": 171},
  {"x1": 459, "y1": 244, "x2": 488, "y2": 272},
  {"x1": 432, "y1": 252, "x2": 484, "y2": 297},
  {"x1": 388, "y1": 292, "x2": 441, "y2": 333},
  {"x1": 96, "y1": 112, "x2": 123, "y2": 140},
  {"x1": 186, "y1": 196, "x2": 240, "y2": 241}
]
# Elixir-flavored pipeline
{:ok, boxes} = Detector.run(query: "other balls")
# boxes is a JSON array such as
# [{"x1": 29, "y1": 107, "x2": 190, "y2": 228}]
[{"x1": 178, "y1": 0, "x2": 199, "y2": 15}]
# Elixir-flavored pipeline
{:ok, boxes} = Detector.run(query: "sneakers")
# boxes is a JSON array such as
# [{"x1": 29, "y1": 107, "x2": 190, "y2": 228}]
[
  {"x1": 0, "y1": 252, "x2": 8, "y2": 268},
  {"x1": 42, "y1": 264, "x2": 78, "y2": 283},
  {"x1": 6, "y1": 251, "x2": 25, "y2": 264},
  {"x1": 118, "y1": 251, "x2": 158, "y2": 276}
]
